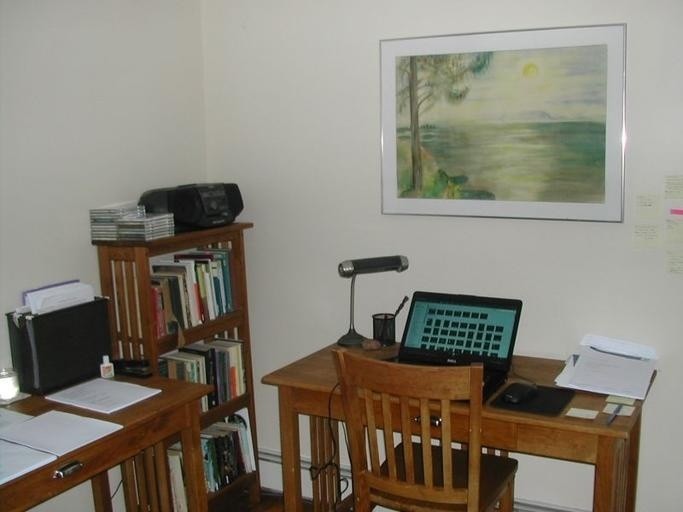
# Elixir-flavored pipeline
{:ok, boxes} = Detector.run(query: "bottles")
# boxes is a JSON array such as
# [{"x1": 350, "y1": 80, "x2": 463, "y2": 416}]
[{"x1": 101, "y1": 354, "x2": 115, "y2": 378}]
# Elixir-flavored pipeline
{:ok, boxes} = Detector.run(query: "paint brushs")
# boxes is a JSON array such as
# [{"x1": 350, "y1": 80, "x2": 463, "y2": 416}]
[{"x1": 381, "y1": 297, "x2": 408, "y2": 337}]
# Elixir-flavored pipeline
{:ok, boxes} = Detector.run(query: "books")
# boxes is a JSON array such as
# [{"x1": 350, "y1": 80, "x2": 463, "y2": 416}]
[
  {"x1": 144, "y1": 248, "x2": 235, "y2": 343},
  {"x1": 165, "y1": 406, "x2": 256, "y2": 511},
  {"x1": 157, "y1": 339, "x2": 246, "y2": 413},
  {"x1": 90, "y1": 205, "x2": 176, "y2": 243}
]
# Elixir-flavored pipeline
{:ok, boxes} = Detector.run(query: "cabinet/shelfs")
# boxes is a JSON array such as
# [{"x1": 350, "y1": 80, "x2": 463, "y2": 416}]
[{"x1": 91, "y1": 219, "x2": 260, "y2": 512}]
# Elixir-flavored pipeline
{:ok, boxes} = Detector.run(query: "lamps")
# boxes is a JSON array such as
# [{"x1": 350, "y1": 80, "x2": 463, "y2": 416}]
[{"x1": 336, "y1": 255, "x2": 409, "y2": 347}]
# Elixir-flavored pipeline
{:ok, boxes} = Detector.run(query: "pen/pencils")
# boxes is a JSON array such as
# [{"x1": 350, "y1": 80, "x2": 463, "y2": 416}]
[{"x1": 608, "y1": 404, "x2": 622, "y2": 426}]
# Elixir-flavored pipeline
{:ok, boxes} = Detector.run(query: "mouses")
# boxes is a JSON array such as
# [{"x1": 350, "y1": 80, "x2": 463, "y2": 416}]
[{"x1": 505, "y1": 383, "x2": 538, "y2": 405}]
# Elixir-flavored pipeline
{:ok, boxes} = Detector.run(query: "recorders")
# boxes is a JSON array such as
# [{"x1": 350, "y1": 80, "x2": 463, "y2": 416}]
[{"x1": 138, "y1": 182, "x2": 244, "y2": 230}]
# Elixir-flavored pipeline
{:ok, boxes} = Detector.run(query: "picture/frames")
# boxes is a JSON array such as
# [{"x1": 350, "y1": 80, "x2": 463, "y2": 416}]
[{"x1": 378, "y1": 21, "x2": 628, "y2": 224}]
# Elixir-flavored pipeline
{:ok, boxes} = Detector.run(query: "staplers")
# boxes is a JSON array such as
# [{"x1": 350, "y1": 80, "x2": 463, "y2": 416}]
[{"x1": 113, "y1": 360, "x2": 152, "y2": 378}]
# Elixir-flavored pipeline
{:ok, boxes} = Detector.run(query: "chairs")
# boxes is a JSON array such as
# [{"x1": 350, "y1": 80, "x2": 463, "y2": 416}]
[{"x1": 330, "y1": 346, "x2": 518, "y2": 512}]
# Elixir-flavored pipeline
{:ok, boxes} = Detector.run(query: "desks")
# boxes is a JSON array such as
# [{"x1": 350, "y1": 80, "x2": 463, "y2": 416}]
[
  {"x1": 262, "y1": 339, "x2": 658, "y2": 511},
  {"x1": 0, "y1": 364, "x2": 214, "y2": 512}
]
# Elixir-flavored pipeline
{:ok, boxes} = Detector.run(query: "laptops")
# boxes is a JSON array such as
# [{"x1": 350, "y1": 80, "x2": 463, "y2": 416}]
[{"x1": 373, "y1": 292, "x2": 523, "y2": 405}]
